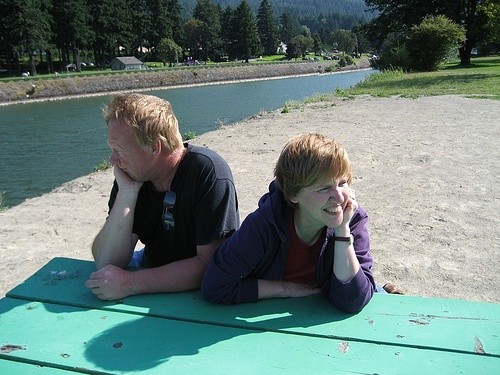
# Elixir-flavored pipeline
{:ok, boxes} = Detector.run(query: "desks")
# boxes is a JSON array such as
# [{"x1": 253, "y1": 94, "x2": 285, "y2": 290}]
[{"x1": 0, "y1": 256, "x2": 499, "y2": 375}]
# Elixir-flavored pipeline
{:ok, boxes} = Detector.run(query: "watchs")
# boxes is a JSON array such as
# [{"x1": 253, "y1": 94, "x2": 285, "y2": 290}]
[{"x1": 332, "y1": 234, "x2": 355, "y2": 244}]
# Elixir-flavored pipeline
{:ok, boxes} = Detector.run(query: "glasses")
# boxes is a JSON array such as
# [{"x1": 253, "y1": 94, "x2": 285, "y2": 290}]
[{"x1": 162, "y1": 191, "x2": 176, "y2": 231}]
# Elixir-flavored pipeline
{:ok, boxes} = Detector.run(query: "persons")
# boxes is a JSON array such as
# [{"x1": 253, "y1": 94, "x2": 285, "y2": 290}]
[
  {"x1": 85, "y1": 93, "x2": 240, "y2": 301},
  {"x1": 198, "y1": 133, "x2": 377, "y2": 315},
  {"x1": 163, "y1": 50, "x2": 378, "y2": 68}
]
